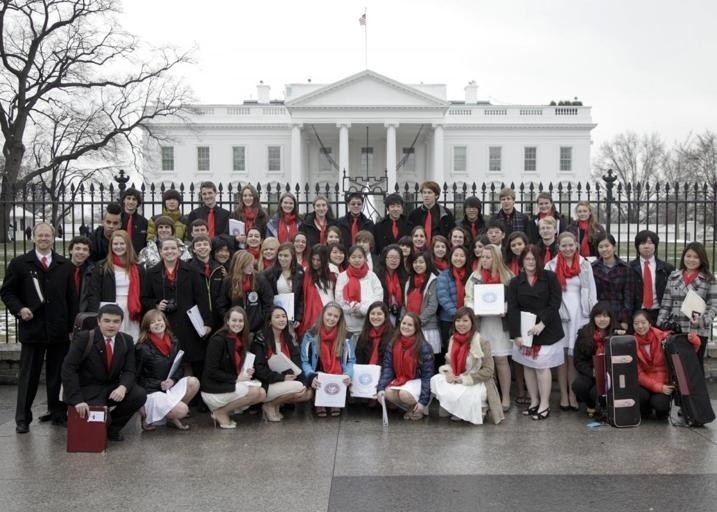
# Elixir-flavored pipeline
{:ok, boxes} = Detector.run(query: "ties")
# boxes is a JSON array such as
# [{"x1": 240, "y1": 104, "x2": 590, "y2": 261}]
[
  {"x1": 42, "y1": 257, "x2": 47, "y2": 269},
  {"x1": 471, "y1": 224, "x2": 476, "y2": 239},
  {"x1": 205, "y1": 263, "x2": 210, "y2": 277},
  {"x1": 544, "y1": 247, "x2": 551, "y2": 265},
  {"x1": 392, "y1": 222, "x2": 399, "y2": 239},
  {"x1": 643, "y1": 261, "x2": 653, "y2": 307},
  {"x1": 208, "y1": 208, "x2": 215, "y2": 240},
  {"x1": 105, "y1": 337, "x2": 113, "y2": 370},
  {"x1": 425, "y1": 210, "x2": 432, "y2": 243},
  {"x1": 74, "y1": 268, "x2": 80, "y2": 297},
  {"x1": 352, "y1": 217, "x2": 358, "y2": 245},
  {"x1": 126, "y1": 214, "x2": 133, "y2": 239}
]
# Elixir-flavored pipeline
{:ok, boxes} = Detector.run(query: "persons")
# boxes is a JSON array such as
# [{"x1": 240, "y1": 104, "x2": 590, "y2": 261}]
[
  {"x1": 1, "y1": 220, "x2": 79, "y2": 434},
  {"x1": 297, "y1": 179, "x2": 637, "y2": 426},
  {"x1": 38, "y1": 178, "x2": 312, "y2": 432},
  {"x1": 627, "y1": 229, "x2": 717, "y2": 427}
]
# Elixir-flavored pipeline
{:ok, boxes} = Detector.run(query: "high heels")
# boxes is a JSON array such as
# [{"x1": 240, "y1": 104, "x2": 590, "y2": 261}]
[
  {"x1": 262, "y1": 403, "x2": 284, "y2": 422},
  {"x1": 210, "y1": 410, "x2": 237, "y2": 429}
]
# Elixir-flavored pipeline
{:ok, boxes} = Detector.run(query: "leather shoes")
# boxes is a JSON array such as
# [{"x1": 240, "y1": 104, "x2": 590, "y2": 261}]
[
  {"x1": 39, "y1": 414, "x2": 52, "y2": 421},
  {"x1": 501, "y1": 404, "x2": 510, "y2": 411},
  {"x1": 16, "y1": 423, "x2": 29, "y2": 434},
  {"x1": 108, "y1": 432, "x2": 124, "y2": 442},
  {"x1": 560, "y1": 405, "x2": 569, "y2": 411},
  {"x1": 569, "y1": 405, "x2": 580, "y2": 411},
  {"x1": 166, "y1": 417, "x2": 190, "y2": 431},
  {"x1": 141, "y1": 415, "x2": 156, "y2": 431},
  {"x1": 586, "y1": 407, "x2": 596, "y2": 417}
]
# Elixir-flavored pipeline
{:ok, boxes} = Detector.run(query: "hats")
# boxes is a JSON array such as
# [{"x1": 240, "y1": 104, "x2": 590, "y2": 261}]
[{"x1": 154, "y1": 216, "x2": 176, "y2": 234}]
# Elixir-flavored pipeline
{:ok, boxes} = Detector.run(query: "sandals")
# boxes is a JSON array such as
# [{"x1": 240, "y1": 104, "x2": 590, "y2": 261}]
[
  {"x1": 403, "y1": 410, "x2": 424, "y2": 421},
  {"x1": 330, "y1": 408, "x2": 342, "y2": 417},
  {"x1": 315, "y1": 406, "x2": 327, "y2": 418},
  {"x1": 532, "y1": 407, "x2": 551, "y2": 420},
  {"x1": 522, "y1": 404, "x2": 539, "y2": 416},
  {"x1": 516, "y1": 396, "x2": 524, "y2": 404},
  {"x1": 526, "y1": 396, "x2": 531, "y2": 403}
]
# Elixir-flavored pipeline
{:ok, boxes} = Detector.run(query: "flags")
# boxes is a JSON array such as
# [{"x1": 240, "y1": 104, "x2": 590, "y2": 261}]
[{"x1": 357, "y1": 12, "x2": 367, "y2": 26}]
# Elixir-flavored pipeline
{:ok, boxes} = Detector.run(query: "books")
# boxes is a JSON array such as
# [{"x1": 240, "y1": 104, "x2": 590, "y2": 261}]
[{"x1": 65, "y1": 404, "x2": 108, "y2": 454}]
